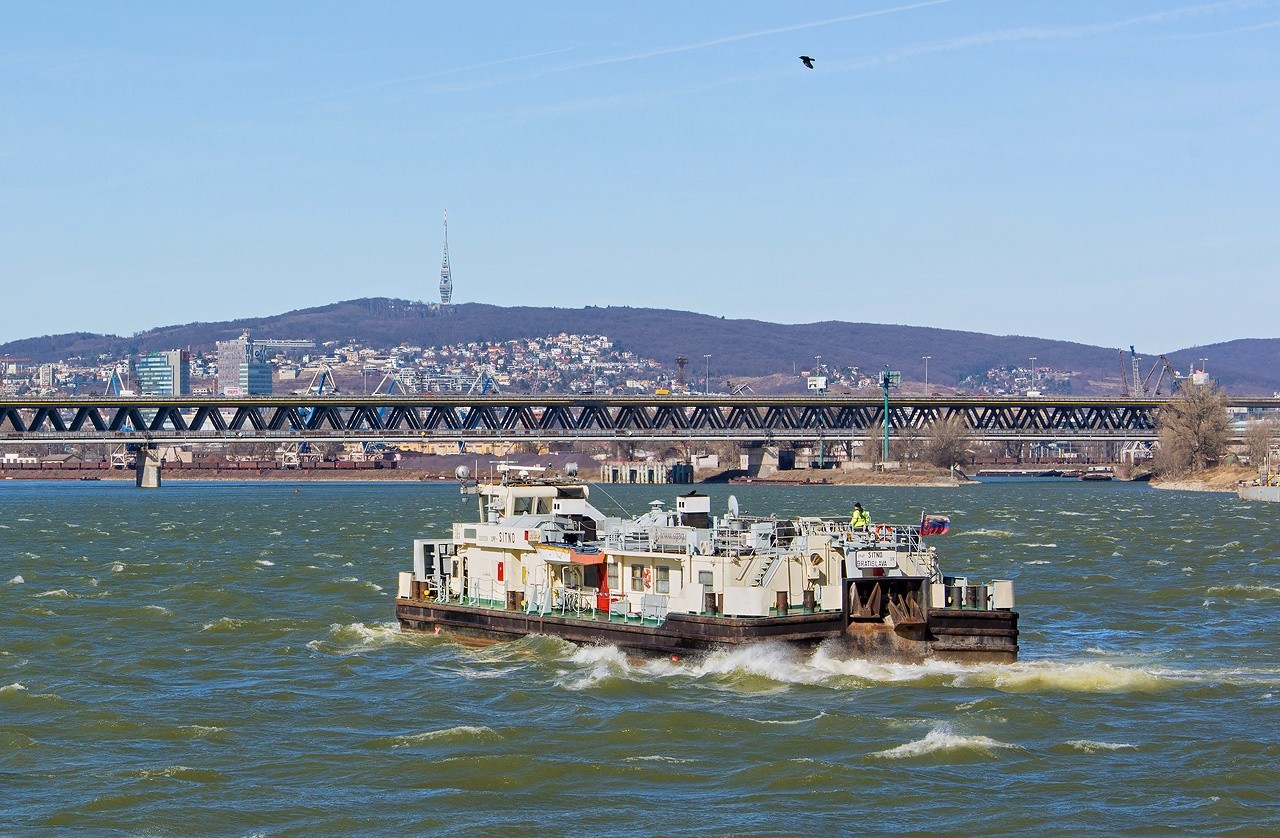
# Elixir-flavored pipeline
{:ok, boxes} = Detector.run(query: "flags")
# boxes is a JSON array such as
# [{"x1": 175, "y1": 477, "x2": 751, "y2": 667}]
[{"x1": 919, "y1": 514, "x2": 951, "y2": 536}]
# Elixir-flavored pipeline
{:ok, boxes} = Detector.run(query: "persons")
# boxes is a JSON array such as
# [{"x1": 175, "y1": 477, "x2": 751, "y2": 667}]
[{"x1": 850, "y1": 502, "x2": 870, "y2": 527}]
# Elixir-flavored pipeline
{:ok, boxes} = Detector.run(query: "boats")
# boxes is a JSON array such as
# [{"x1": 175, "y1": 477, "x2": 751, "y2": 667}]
[{"x1": 397, "y1": 467, "x2": 1019, "y2": 667}]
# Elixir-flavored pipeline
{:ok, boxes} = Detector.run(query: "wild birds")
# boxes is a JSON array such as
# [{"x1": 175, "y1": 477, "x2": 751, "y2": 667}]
[{"x1": 799, "y1": 55, "x2": 815, "y2": 69}]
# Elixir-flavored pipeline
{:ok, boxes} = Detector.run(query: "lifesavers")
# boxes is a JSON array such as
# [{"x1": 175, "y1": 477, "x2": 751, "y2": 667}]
[
  {"x1": 874, "y1": 523, "x2": 893, "y2": 543},
  {"x1": 641, "y1": 567, "x2": 652, "y2": 589}
]
